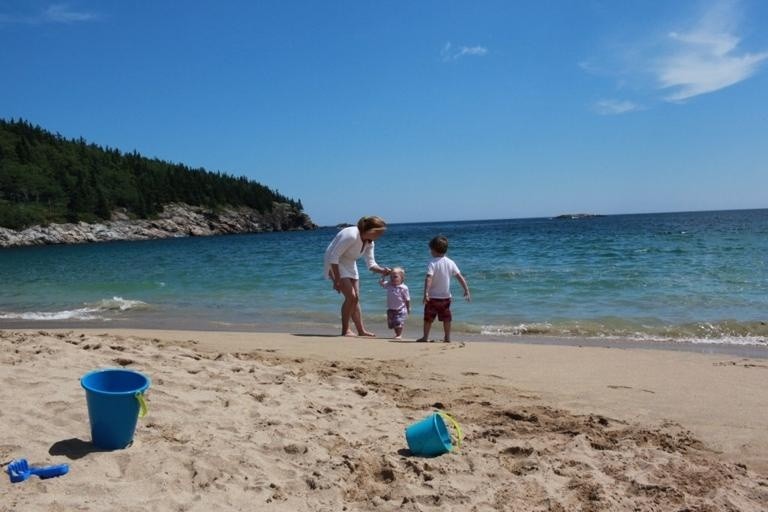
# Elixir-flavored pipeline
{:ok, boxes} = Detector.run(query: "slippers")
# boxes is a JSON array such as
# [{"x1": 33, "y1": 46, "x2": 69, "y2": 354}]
[{"x1": 8, "y1": 458, "x2": 69, "y2": 483}]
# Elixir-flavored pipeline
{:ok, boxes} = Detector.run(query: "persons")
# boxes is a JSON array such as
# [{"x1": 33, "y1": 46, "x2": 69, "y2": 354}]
[
  {"x1": 378, "y1": 267, "x2": 412, "y2": 340},
  {"x1": 324, "y1": 215, "x2": 390, "y2": 337},
  {"x1": 416, "y1": 235, "x2": 471, "y2": 342}
]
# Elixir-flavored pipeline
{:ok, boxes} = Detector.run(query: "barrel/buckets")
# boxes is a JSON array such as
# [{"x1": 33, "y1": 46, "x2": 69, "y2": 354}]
[
  {"x1": 405, "y1": 411, "x2": 464, "y2": 458},
  {"x1": 79, "y1": 368, "x2": 151, "y2": 451}
]
